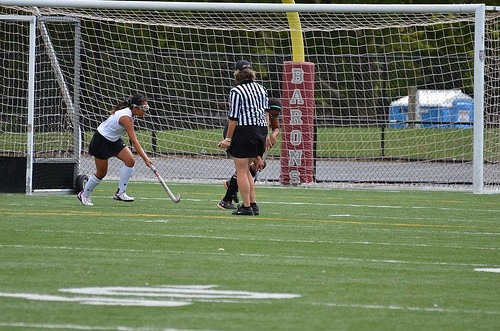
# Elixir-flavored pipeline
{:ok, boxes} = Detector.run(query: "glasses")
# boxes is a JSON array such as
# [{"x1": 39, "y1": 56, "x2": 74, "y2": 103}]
[
  {"x1": 269, "y1": 113, "x2": 279, "y2": 117},
  {"x1": 133, "y1": 104, "x2": 150, "y2": 111}
]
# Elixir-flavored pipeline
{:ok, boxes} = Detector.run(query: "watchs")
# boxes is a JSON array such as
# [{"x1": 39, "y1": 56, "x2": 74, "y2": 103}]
[{"x1": 226, "y1": 136, "x2": 232, "y2": 142}]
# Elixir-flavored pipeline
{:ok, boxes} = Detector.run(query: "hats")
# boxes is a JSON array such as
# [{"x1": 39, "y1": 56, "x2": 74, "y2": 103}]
[{"x1": 236, "y1": 60, "x2": 250, "y2": 69}]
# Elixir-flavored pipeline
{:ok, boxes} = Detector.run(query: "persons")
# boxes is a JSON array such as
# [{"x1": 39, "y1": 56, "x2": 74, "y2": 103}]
[
  {"x1": 218, "y1": 98, "x2": 281, "y2": 211},
  {"x1": 218, "y1": 59, "x2": 272, "y2": 215},
  {"x1": 76, "y1": 94, "x2": 156, "y2": 206}
]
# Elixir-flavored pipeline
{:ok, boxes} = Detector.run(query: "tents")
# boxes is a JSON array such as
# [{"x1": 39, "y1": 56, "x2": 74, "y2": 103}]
[{"x1": 388, "y1": 89, "x2": 473, "y2": 129}]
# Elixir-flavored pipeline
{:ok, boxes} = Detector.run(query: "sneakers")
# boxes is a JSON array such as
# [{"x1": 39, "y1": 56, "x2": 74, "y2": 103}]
[
  {"x1": 252, "y1": 206, "x2": 259, "y2": 215},
  {"x1": 114, "y1": 192, "x2": 134, "y2": 201},
  {"x1": 224, "y1": 180, "x2": 239, "y2": 203},
  {"x1": 217, "y1": 198, "x2": 237, "y2": 209},
  {"x1": 232, "y1": 207, "x2": 254, "y2": 215},
  {"x1": 77, "y1": 190, "x2": 94, "y2": 205}
]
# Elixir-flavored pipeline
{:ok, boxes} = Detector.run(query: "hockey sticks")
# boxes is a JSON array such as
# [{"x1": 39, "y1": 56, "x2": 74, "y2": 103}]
[
  {"x1": 150, "y1": 162, "x2": 180, "y2": 203},
  {"x1": 237, "y1": 150, "x2": 268, "y2": 210}
]
